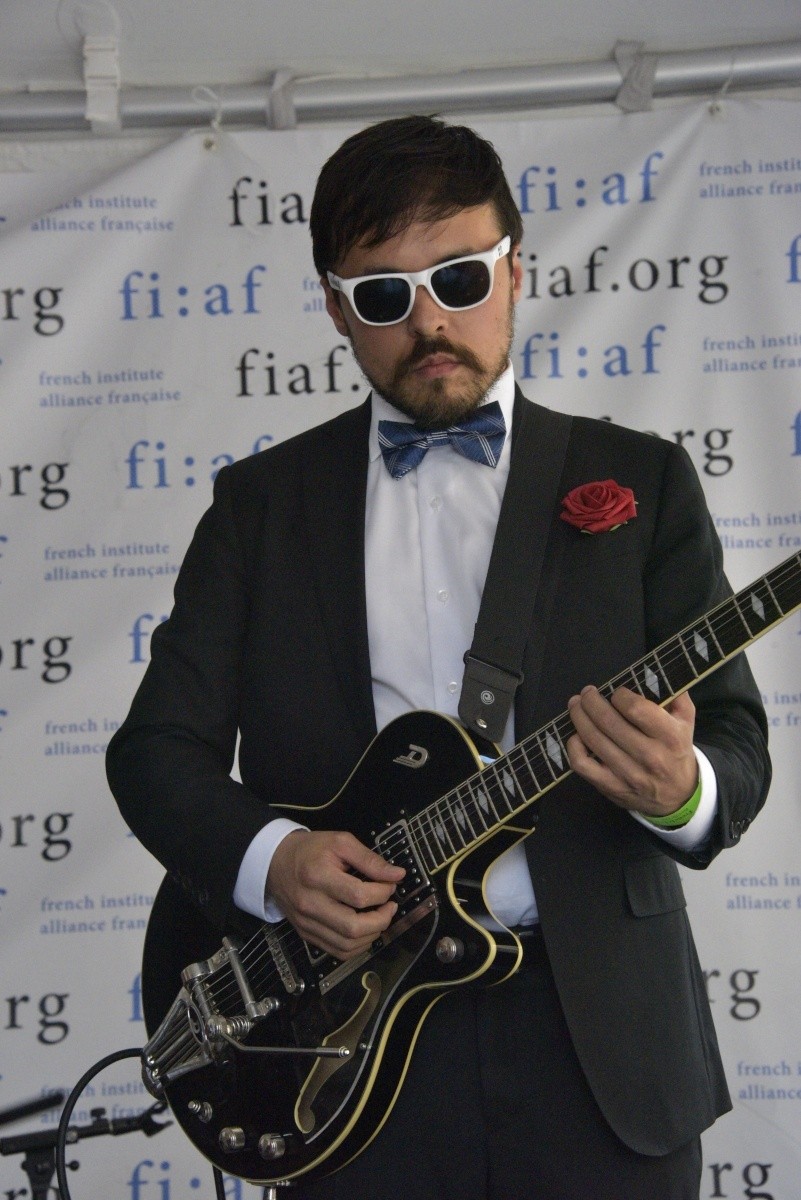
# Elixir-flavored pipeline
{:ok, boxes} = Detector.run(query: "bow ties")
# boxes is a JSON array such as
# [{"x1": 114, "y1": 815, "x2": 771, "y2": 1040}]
[{"x1": 378, "y1": 399, "x2": 506, "y2": 481}]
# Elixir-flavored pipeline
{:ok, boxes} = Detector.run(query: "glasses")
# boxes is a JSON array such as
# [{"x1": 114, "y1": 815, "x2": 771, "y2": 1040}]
[{"x1": 327, "y1": 235, "x2": 511, "y2": 326}]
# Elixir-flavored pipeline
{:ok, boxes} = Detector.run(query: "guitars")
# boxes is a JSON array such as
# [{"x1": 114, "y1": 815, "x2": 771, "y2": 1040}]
[{"x1": 140, "y1": 550, "x2": 801, "y2": 1190}]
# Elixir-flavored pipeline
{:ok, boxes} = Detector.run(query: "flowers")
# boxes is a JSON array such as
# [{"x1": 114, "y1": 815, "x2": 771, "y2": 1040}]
[{"x1": 563, "y1": 479, "x2": 640, "y2": 536}]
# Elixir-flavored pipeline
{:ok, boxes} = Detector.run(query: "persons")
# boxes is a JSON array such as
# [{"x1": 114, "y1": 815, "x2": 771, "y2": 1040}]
[{"x1": 105, "y1": 113, "x2": 773, "y2": 1200}]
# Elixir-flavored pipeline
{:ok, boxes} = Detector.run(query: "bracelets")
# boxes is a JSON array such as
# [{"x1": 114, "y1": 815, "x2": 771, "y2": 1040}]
[{"x1": 638, "y1": 767, "x2": 701, "y2": 827}]
